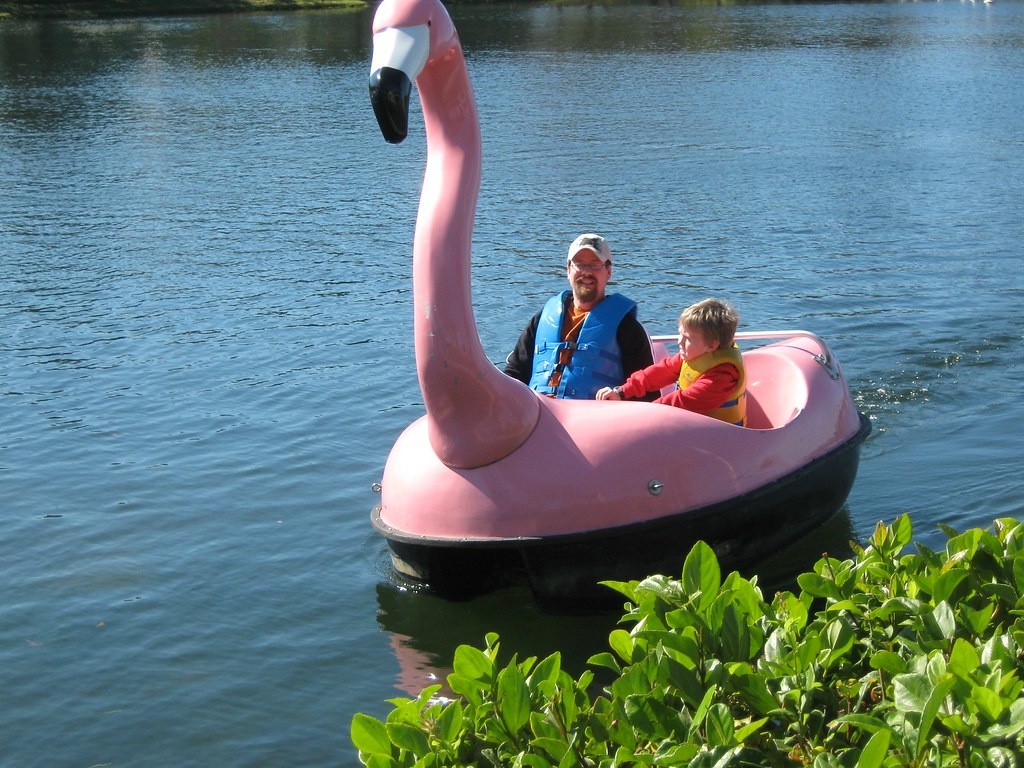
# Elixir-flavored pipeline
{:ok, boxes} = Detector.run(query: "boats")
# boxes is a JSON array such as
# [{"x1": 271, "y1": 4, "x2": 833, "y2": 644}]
[{"x1": 362, "y1": 0, "x2": 872, "y2": 581}]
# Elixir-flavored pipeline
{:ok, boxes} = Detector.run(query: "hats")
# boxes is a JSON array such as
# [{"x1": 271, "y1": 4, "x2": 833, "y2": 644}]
[{"x1": 570, "y1": 233, "x2": 611, "y2": 267}]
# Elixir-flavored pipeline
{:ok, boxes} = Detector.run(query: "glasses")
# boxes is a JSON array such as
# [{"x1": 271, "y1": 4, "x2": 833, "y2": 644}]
[{"x1": 568, "y1": 262, "x2": 607, "y2": 271}]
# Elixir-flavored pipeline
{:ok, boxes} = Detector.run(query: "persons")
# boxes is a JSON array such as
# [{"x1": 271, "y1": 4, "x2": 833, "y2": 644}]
[
  {"x1": 596, "y1": 298, "x2": 748, "y2": 428},
  {"x1": 503, "y1": 233, "x2": 662, "y2": 403}
]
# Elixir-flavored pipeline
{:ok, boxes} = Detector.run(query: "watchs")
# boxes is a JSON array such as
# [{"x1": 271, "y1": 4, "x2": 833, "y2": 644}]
[{"x1": 613, "y1": 386, "x2": 625, "y2": 401}]
